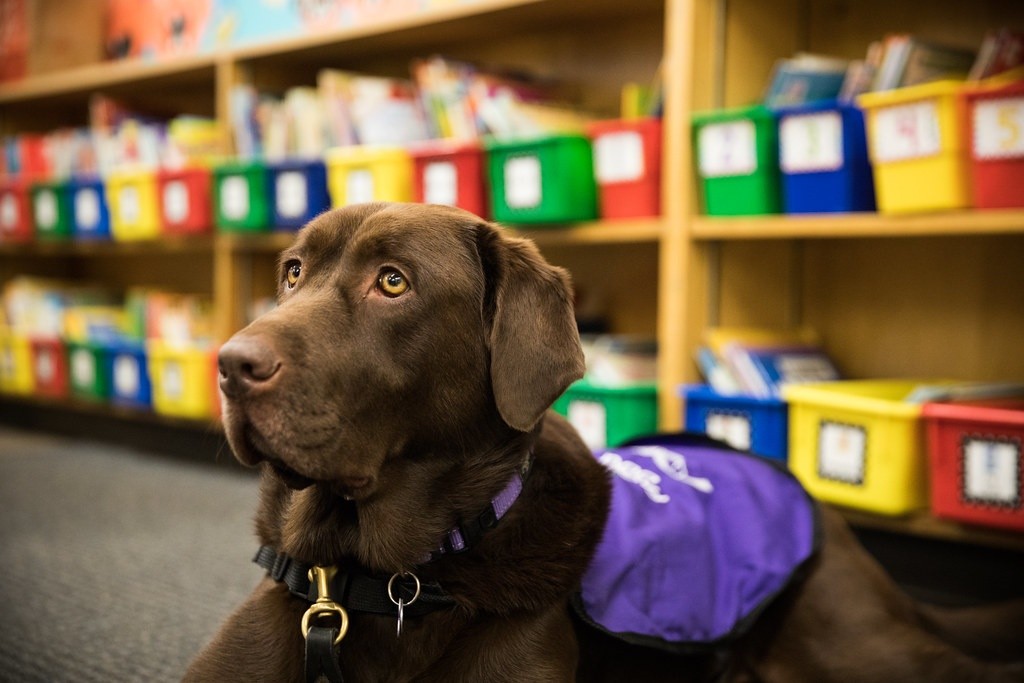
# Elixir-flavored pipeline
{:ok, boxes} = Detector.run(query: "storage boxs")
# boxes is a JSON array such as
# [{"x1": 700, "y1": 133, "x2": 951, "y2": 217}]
[
  {"x1": 682, "y1": 377, "x2": 1024, "y2": 535},
  {"x1": 0, "y1": 113, "x2": 660, "y2": 453},
  {"x1": 690, "y1": 72, "x2": 1024, "y2": 217}
]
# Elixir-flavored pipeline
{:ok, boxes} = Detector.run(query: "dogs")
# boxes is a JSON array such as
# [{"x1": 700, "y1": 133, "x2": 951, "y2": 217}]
[{"x1": 180, "y1": 202, "x2": 1024, "y2": 683}]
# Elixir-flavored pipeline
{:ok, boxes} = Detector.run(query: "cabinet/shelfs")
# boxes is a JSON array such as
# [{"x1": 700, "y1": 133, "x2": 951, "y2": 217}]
[{"x1": 1, "y1": 0, "x2": 1024, "y2": 542}]
